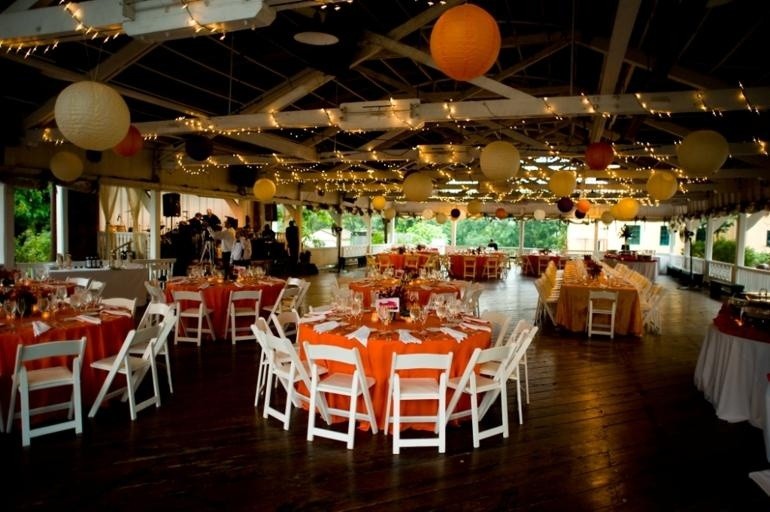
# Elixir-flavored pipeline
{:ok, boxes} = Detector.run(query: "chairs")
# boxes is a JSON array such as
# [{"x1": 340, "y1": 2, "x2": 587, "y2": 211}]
[
  {"x1": 88, "y1": 321, "x2": 167, "y2": 421},
  {"x1": 365, "y1": 253, "x2": 377, "y2": 277},
  {"x1": 170, "y1": 288, "x2": 215, "y2": 347},
  {"x1": 421, "y1": 254, "x2": 438, "y2": 274},
  {"x1": 5, "y1": 336, "x2": 88, "y2": 447},
  {"x1": 224, "y1": 288, "x2": 262, "y2": 345},
  {"x1": 285, "y1": 276, "x2": 311, "y2": 310},
  {"x1": 302, "y1": 340, "x2": 379, "y2": 451},
  {"x1": 377, "y1": 255, "x2": 395, "y2": 275},
  {"x1": 144, "y1": 279, "x2": 166, "y2": 303},
  {"x1": 463, "y1": 282, "x2": 483, "y2": 314},
  {"x1": 128, "y1": 297, "x2": 177, "y2": 394},
  {"x1": 585, "y1": 288, "x2": 619, "y2": 339},
  {"x1": 463, "y1": 254, "x2": 477, "y2": 279},
  {"x1": 537, "y1": 256, "x2": 550, "y2": 276},
  {"x1": 521, "y1": 256, "x2": 534, "y2": 275},
  {"x1": 257, "y1": 330, "x2": 329, "y2": 431},
  {"x1": 88, "y1": 281, "x2": 105, "y2": 298},
  {"x1": 146, "y1": 257, "x2": 176, "y2": 281},
  {"x1": 614, "y1": 263, "x2": 664, "y2": 335},
  {"x1": 482, "y1": 257, "x2": 498, "y2": 280},
  {"x1": 263, "y1": 285, "x2": 304, "y2": 332},
  {"x1": 383, "y1": 351, "x2": 454, "y2": 455},
  {"x1": 65, "y1": 277, "x2": 91, "y2": 288},
  {"x1": 462, "y1": 289, "x2": 480, "y2": 318},
  {"x1": 270, "y1": 309, "x2": 300, "y2": 351},
  {"x1": 534, "y1": 261, "x2": 564, "y2": 326},
  {"x1": 404, "y1": 254, "x2": 419, "y2": 268},
  {"x1": 479, "y1": 320, "x2": 539, "y2": 424},
  {"x1": 444, "y1": 342, "x2": 518, "y2": 448},
  {"x1": 480, "y1": 308, "x2": 512, "y2": 347},
  {"x1": 454, "y1": 278, "x2": 472, "y2": 291},
  {"x1": 254, "y1": 316, "x2": 299, "y2": 406},
  {"x1": 98, "y1": 295, "x2": 137, "y2": 316},
  {"x1": 493, "y1": 256, "x2": 509, "y2": 280},
  {"x1": 558, "y1": 256, "x2": 570, "y2": 269}
]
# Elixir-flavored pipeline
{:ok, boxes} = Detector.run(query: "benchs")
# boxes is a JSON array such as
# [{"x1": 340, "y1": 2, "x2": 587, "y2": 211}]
[
  {"x1": 708, "y1": 278, "x2": 744, "y2": 301},
  {"x1": 340, "y1": 256, "x2": 366, "y2": 270}
]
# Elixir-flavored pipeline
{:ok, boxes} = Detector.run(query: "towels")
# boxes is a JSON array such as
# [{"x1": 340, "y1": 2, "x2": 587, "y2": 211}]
[
  {"x1": 465, "y1": 316, "x2": 490, "y2": 324},
  {"x1": 300, "y1": 316, "x2": 326, "y2": 322},
  {"x1": 458, "y1": 320, "x2": 491, "y2": 334},
  {"x1": 104, "y1": 310, "x2": 131, "y2": 318},
  {"x1": 345, "y1": 324, "x2": 371, "y2": 348},
  {"x1": 76, "y1": 313, "x2": 101, "y2": 325},
  {"x1": 397, "y1": 328, "x2": 419, "y2": 346},
  {"x1": 198, "y1": 284, "x2": 210, "y2": 290},
  {"x1": 312, "y1": 320, "x2": 339, "y2": 334},
  {"x1": 439, "y1": 324, "x2": 467, "y2": 343},
  {"x1": 256, "y1": 280, "x2": 273, "y2": 286},
  {"x1": 232, "y1": 281, "x2": 244, "y2": 288},
  {"x1": 311, "y1": 305, "x2": 332, "y2": 314},
  {"x1": 32, "y1": 319, "x2": 52, "y2": 336},
  {"x1": 175, "y1": 280, "x2": 189, "y2": 286}
]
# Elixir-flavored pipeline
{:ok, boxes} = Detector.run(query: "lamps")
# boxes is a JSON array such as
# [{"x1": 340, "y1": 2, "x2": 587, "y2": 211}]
[{"x1": 295, "y1": 11, "x2": 337, "y2": 45}]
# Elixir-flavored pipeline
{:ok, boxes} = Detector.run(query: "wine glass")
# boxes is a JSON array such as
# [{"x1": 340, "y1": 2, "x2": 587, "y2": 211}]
[
  {"x1": 186, "y1": 264, "x2": 207, "y2": 282},
  {"x1": 332, "y1": 288, "x2": 389, "y2": 331},
  {"x1": 1, "y1": 279, "x2": 103, "y2": 333},
  {"x1": 365, "y1": 261, "x2": 395, "y2": 282},
  {"x1": 236, "y1": 266, "x2": 264, "y2": 283},
  {"x1": 418, "y1": 267, "x2": 443, "y2": 283},
  {"x1": 409, "y1": 291, "x2": 469, "y2": 331}
]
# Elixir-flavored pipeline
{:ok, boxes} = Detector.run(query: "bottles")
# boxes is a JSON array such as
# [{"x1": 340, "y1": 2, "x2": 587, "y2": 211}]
[
  {"x1": 85, "y1": 256, "x2": 91, "y2": 268},
  {"x1": 90, "y1": 256, "x2": 95, "y2": 269},
  {"x1": 94, "y1": 256, "x2": 100, "y2": 268},
  {"x1": 120, "y1": 246, "x2": 136, "y2": 260}
]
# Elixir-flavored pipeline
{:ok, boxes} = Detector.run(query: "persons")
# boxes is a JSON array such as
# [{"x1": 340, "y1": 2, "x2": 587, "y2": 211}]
[
  {"x1": 159, "y1": 208, "x2": 319, "y2": 282},
  {"x1": 488, "y1": 239, "x2": 498, "y2": 251}
]
